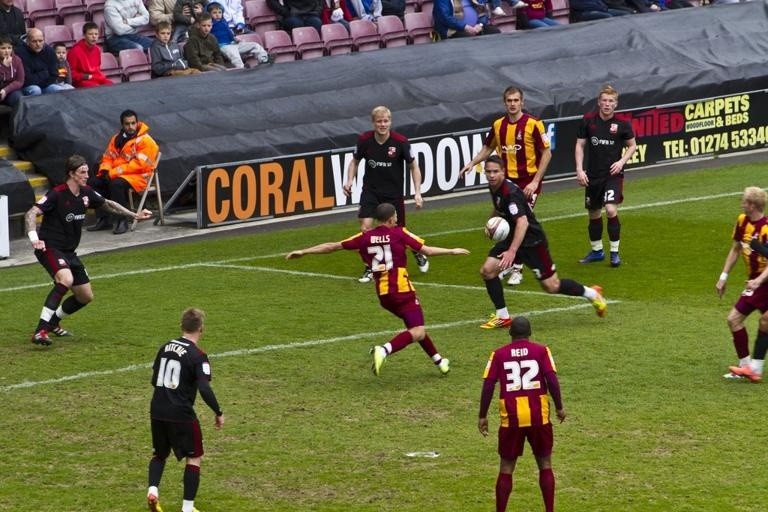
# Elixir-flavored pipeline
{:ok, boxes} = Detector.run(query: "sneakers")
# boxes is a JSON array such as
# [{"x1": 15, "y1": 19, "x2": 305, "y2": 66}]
[
  {"x1": 479, "y1": 313, "x2": 513, "y2": 330},
  {"x1": 589, "y1": 285, "x2": 607, "y2": 318},
  {"x1": 577, "y1": 249, "x2": 606, "y2": 263},
  {"x1": 147, "y1": 493, "x2": 163, "y2": 512},
  {"x1": 358, "y1": 269, "x2": 374, "y2": 284},
  {"x1": 610, "y1": 252, "x2": 620, "y2": 267},
  {"x1": 722, "y1": 366, "x2": 763, "y2": 383},
  {"x1": 48, "y1": 323, "x2": 72, "y2": 337},
  {"x1": 369, "y1": 346, "x2": 385, "y2": 377},
  {"x1": 32, "y1": 328, "x2": 52, "y2": 346},
  {"x1": 180, "y1": 507, "x2": 205, "y2": 512},
  {"x1": 440, "y1": 359, "x2": 450, "y2": 374},
  {"x1": 414, "y1": 253, "x2": 430, "y2": 273},
  {"x1": 507, "y1": 270, "x2": 523, "y2": 285}
]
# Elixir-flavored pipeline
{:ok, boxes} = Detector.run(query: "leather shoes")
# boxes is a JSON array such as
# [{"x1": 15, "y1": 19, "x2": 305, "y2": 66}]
[
  {"x1": 88, "y1": 217, "x2": 113, "y2": 231},
  {"x1": 114, "y1": 220, "x2": 128, "y2": 234}
]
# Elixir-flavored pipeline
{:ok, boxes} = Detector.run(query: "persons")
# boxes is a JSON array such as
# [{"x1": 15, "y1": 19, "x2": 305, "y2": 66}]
[
  {"x1": 149, "y1": 2, "x2": 278, "y2": 72},
  {"x1": 716, "y1": 186, "x2": 768, "y2": 379},
  {"x1": 266, "y1": 2, "x2": 405, "y2": 35},
  {"x1": 729, "y1": 235, "x2": 767, "y2": 383},
  {"x1": 1, "y1": 2, "x2": 26, "y2": 38},
  {"x1": 15, "y1": 28, "x2": 63, "y2": 96},
  {"x1": 103, "y1": 2, "x2": 155, "y2": 55},
  {"x1": 576, "y1": 86, "x2": 637, "y2": 267},
  {"x1": 343, "y1": 106, "x2": 430, "y2": 282},
  {"x1": 432, "y1": 0, "x2": 696, "y2": 40},
  {"x1": 479, "y1": 316, "x2": 565, "y2": 511},
  {"x1": 24, "y1": 155, "x2": 152, "y2": 344},
  {"x1": 66, "y1": 22, "x2": 116, "y2": 87},
  {"x1": 54, "y1": 42, "x2": 74, "y2": 90},
  {"x1": 459, "y1": 86, "x2": 552, "y2": 285},
  {"x1": 1, "y1": 34, "x2": 26, "y2": 104},
  {"x1": 285, "y1": 204, "x2": 471, "y2": 374},
  {"x1": 147, "y1": 308, "x2": 224, "y2": 512},
  {"x1": 87, "y1": 111, "x2": 158, "y2": 233},
  {"x1": 478, "y1": 155, "x2": 607, "y2": 330},
  {"x1": 151, "y1": 22, "x2": 200, "y2": 79}
]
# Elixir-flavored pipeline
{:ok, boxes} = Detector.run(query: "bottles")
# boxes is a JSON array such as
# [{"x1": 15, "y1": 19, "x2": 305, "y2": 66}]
[
  {"x1": 234, "y1": 23, "x2": 251, "y2": 32},
  {"x1": 473, "y1": 22, "x2": 482, "y2": 34}
]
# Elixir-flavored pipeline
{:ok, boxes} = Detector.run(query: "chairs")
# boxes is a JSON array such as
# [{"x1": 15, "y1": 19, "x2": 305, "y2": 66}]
[
  {"x1": 13, "y1": 0, "x2": 570, "y2": 84},
  {"x1": 126, "y1": 151, "x2": 164, "y2": 231}
]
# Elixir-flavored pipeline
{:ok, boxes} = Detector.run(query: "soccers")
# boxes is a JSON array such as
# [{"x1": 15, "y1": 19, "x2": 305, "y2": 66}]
[{"x1": 484, "y1": 216, "x2": 510, "y2": 242}]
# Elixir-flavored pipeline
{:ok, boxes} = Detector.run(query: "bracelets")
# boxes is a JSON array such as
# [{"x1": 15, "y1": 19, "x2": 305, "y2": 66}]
[
  {"x1": 718, "y1": 272, "x2": 729, "y2": 282},
  {"x1": 27, "y1": 231, "x2": 39, "y2": 243}
]
